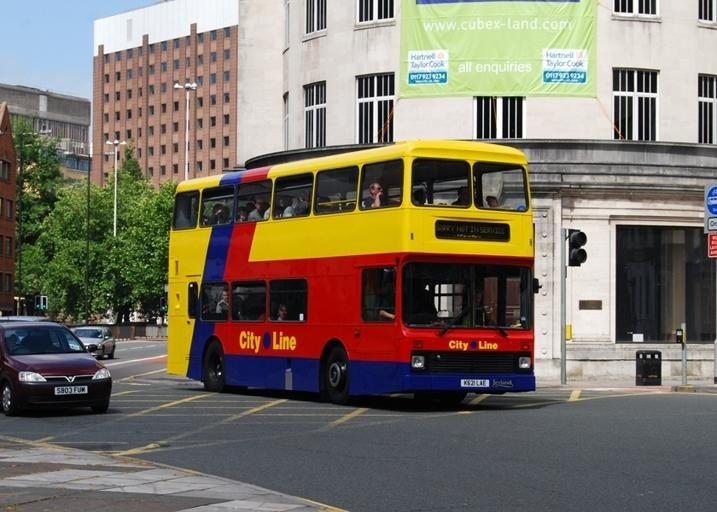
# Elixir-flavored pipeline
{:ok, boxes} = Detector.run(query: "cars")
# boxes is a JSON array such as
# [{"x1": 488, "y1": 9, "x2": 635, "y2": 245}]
[
  {"x1": 54, "y1": 325, "x2": 116, "y2": 360},
  {"x1": 0, "y1": 320, "x2": 113, "y2": 416}
]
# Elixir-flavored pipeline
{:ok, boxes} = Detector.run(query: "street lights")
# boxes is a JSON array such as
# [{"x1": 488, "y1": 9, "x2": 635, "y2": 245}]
[
  {"x1": 171, "y1": 81, "x2": 199, "y2": 183},
  {"x1": 105, "y1": 139, "x2": 128, "y2": 239}
]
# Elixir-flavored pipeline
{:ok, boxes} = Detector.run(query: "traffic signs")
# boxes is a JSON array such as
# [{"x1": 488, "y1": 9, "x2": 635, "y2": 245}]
[
  {"x1": 704, "y1": 182, "x2": 717, "y2": 234},
  {"x1": 707, "y1": 231, "x2": 717, "y2": 258}
]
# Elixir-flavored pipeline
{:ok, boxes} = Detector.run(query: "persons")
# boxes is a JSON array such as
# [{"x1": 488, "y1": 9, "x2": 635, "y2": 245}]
[
  {"x1": 451, "y1": 186, "x2": 469, "y2": 205},
  {"x1": 9, "y1": 334, "x2": 18, "y2": 350},
  {"x1": 414, "y1": 188, "x2": 426, "y2": 206},
  {"x1": 486, "y1": 196, "x2": 499, "y2": 207},
  {"x1": 278, "y1": 304, "x2": 288, "y2": 321},
  {"x1": 216, "y1": 289, "x2": 229, "y2": 313},
  {"x1": 273, "y1": 191, "x2": 311, "y2": 218},
  {"x1": 362, "y1": 183, "x2": 384, "y2": 208},
  {"x1": 194, "y1": 201, "x2": 231, "y2": 225},
  {"x1": 237, "y1": 196, "x2": 270, "y2": 223}
]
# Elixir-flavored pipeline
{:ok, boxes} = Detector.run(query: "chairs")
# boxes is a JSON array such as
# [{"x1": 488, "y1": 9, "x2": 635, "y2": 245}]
[{"x1": 202, "y1": 200, "x2": 366, "y2": 225}]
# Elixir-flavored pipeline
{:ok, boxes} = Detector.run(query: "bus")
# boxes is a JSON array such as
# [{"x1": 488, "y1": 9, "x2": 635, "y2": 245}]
[{"x1": 162, "y1": 138, "x2": 544, "y2": 413}]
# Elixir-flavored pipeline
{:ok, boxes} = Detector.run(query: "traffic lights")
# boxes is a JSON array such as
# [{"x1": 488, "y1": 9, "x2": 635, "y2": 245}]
[{"x1": 566, "y1": 228, "x2": 588, "y2": 269}]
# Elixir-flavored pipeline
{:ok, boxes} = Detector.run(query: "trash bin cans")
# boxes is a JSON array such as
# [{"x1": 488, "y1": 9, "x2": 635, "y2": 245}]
[{"x1": 636, "y1": 350, "x2": 661, "y2": 386}]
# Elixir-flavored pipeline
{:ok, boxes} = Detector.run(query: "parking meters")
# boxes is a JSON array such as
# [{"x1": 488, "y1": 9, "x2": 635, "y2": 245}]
[{"x1": 674, "y1": 321, "x2": 688, "y2": 385}]
